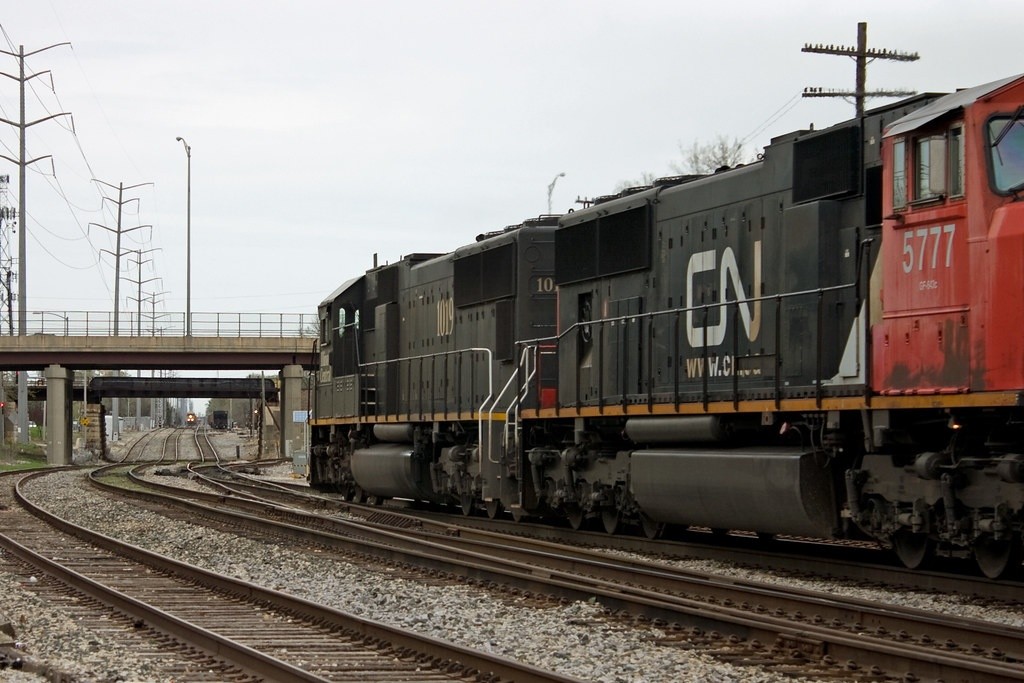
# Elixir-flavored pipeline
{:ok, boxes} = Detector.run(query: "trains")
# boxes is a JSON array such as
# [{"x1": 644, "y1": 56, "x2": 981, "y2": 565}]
[
  {"x1": 186, "y1": 413, "x2": 197, "y2": 426},
  {"x1": 308, "y1": 73, "x2": 1024, "y2": 580},
  {"x1": 208, "y1": 411, "x2": 228, "y2": 429}
]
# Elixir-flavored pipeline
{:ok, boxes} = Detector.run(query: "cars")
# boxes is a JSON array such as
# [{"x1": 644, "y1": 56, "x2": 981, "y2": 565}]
[
  {"x1": 29, "y1": 421, "x2": 37, "y2": 428},
  {"x1": 73, "y1": 420, "x2": 80, "y2": 433}
]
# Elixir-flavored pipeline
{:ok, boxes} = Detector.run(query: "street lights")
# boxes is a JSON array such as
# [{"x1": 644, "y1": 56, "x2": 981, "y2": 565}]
[
  {"x1": 547, "y1": 173, "x2": 564, "y2": 214},
  {"x1": 33, "y1": 312, "x2": 68, "y2": 336},
  {"x1": 177, "y1": 136, "x2": 191, "y2": 336}
]
